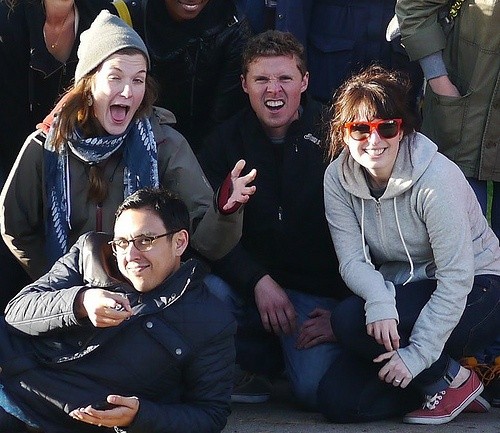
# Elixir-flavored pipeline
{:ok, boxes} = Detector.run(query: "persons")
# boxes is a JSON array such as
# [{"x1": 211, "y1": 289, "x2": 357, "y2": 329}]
[
  {"x1": 323, "y1": 65, "x2": 500, "y2": 424},
  {"x1": 195, "y1": 31, "x2": 376, "y2": 407},
  {"x1": 235, "y1": 0, "x2": 419, "y2": 104},
  {"x1": 102, "y1": 0, "x2": 254, "y2": 151},
  {"x1": 0, "y1": 186, "x2": 237, "y2": 433},
  {"x1": 0, "y1": 9, "x2": 271, "y2": 404},
  {"x1": 0, "y1": 0, "x2": 112, "y2": 193},
  {"x1": 394, "y1": 0, "x2": 500, "y2": 236}
]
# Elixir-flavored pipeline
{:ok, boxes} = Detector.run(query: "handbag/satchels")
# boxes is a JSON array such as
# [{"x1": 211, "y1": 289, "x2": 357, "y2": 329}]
[{"x1": 385, "y1": 0, "x2": 465, "y2": 56}]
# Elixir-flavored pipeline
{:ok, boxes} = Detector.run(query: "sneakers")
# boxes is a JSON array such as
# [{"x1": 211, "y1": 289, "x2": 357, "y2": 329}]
[
  {"x1": 462, "y1": 395, "x2": 491, "y2": 413},
  {"x1": 402, "y1": 368, "x2": 484, "y2": 425}
]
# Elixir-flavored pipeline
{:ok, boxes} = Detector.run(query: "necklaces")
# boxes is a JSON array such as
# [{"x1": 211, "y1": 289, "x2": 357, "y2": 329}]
[{"x1": 46, "y1": 12, "x2": 70, "y2": 48}]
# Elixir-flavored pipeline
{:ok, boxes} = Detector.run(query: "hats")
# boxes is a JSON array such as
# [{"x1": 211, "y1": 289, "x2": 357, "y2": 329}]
[{"x1": 74, "y1": 9, "x2": 151, "y2": 84}]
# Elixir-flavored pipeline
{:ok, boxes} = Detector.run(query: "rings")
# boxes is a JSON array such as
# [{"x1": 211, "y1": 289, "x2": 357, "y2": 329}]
[{"x1": 98, "y1": 423, "x2": 102, "y2": 426}]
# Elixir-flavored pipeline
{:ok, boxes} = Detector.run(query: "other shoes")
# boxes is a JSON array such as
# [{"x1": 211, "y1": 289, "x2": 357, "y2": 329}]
[{"x1": 232, "y1": 370, "x2": 274, "y2": 404}]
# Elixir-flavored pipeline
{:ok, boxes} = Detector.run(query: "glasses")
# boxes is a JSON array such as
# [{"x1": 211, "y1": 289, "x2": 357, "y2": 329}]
[
  {"x1": 345, "y1": 118, "x2": 402, "y2": 141},
  {"x1": 108, "y1": 229, "x2": 181, "y2": 255}
]
